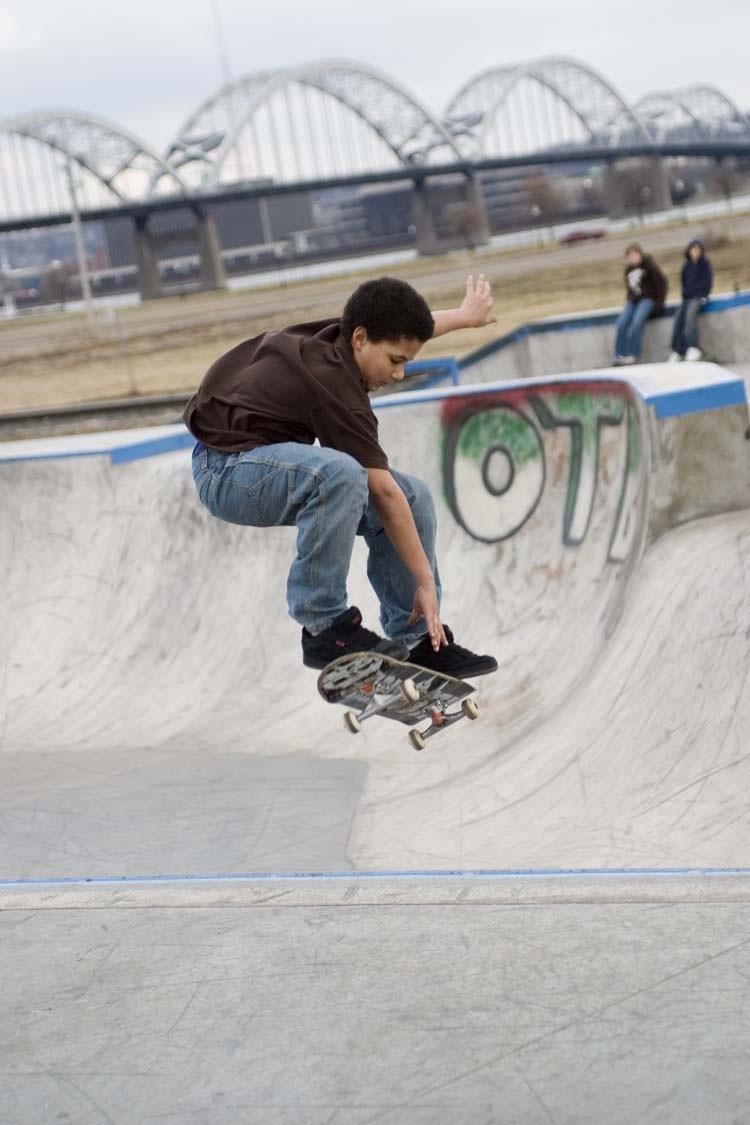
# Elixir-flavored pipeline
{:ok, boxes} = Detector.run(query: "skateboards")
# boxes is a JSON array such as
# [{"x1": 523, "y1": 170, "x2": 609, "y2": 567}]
[{"x1": 317, "y1": 650, "x2": 479, "y2": 752}]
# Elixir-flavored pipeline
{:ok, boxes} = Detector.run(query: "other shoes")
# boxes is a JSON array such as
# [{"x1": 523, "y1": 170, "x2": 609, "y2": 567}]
[
  {"x1": 612, "y1": 355, "x2": 639, "y2": 367},
  {"x1": 668, "y1": 351, "x2": 684, "y2": 362},
  {"x1": 685, "y1": 346, "x2": 702, "y2": 360}
]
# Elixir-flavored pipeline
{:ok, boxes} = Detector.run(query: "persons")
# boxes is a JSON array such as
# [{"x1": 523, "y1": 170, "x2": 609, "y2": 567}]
[
  {"x1": 613, "y1": 243, "x2": 667, "y2": 368},
  {"x1": 667, "y1": 240, "x2": 712, "y2": 362},
  {"x1": 184, "y1": 269, "x2": 499, "y2": 680}
]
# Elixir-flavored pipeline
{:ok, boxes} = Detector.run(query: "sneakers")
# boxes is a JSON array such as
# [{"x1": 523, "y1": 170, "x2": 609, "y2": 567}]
[
  {"x1": 303, "y1": 605, "x2": 411, "y2": 672},
  {"x1": 405, "y1": 622, "x2": 498, "y2": 680}
]
